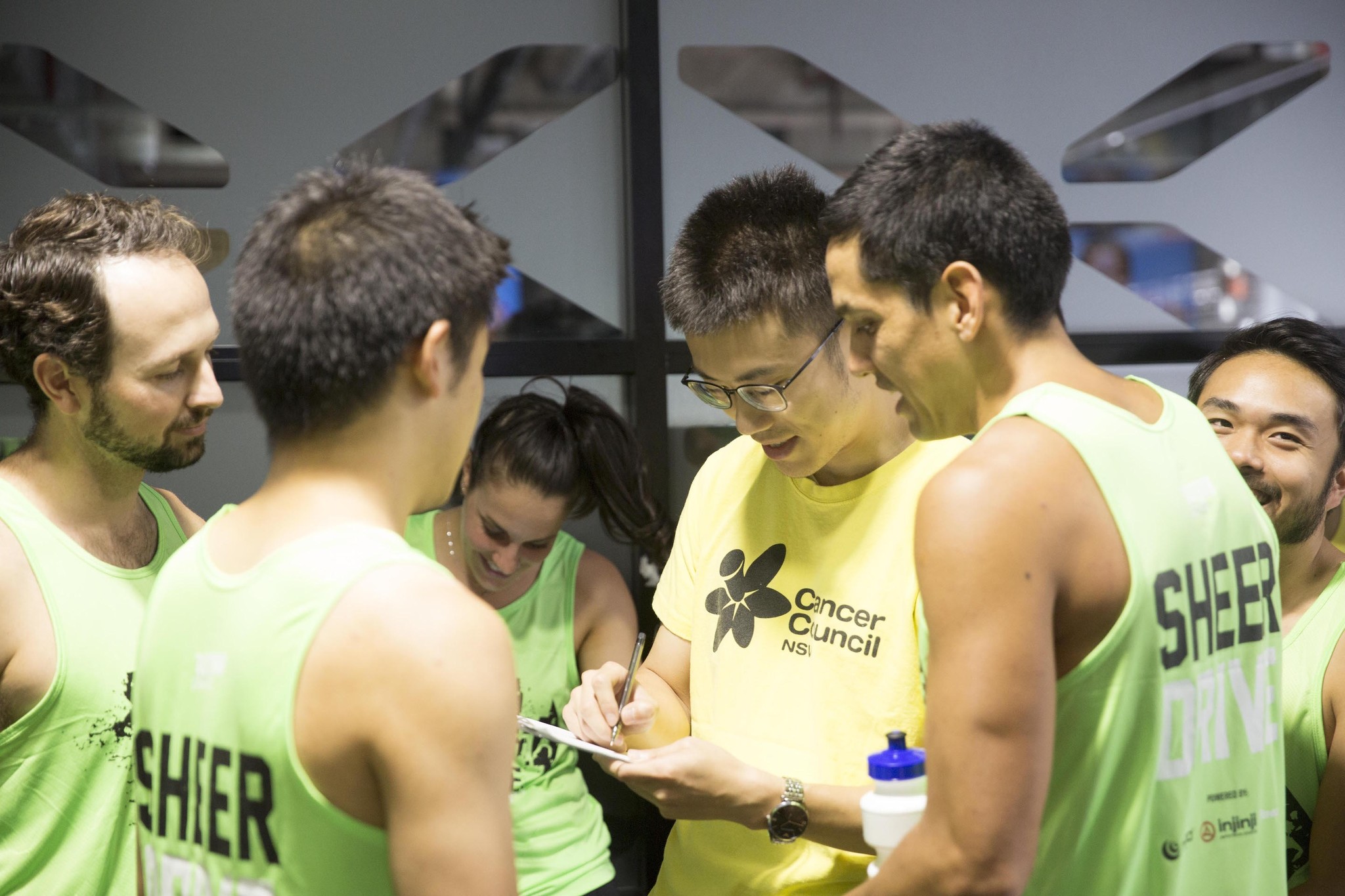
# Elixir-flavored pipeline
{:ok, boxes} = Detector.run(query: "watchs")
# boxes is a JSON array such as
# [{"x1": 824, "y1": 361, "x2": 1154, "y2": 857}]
[{"x1": 768, "y1": 775, "x2": 808, "y2": 845}]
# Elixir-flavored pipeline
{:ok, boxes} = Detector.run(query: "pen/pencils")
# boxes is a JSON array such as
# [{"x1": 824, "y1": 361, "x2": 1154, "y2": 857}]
[{"x1": 610, "y1": 632, "x2": 645, "y2": 745}]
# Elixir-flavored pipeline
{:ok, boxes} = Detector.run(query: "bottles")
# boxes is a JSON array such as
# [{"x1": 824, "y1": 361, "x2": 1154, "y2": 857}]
[{"x1": 861, "y1": 732, "x2": 926, "y2": 879}]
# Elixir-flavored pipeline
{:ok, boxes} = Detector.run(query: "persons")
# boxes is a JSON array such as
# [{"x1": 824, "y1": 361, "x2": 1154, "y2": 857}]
[
  {"x1": 129, "y1": 166, "x2": 516, "y2": 896},
  {"x1": 820, "y1": 118, "x2": 1287, "y2": 896},
  {"x1": 402, "y1": 383, "x2": 668, "y2": 896},
  {"x1": 0, "y1": 197, "x2": 225, "y2": 896},
  {"x1": 563, "y1": 164, "x2": 975, "y2": 896},
  {"x1": 1188, "y1": 317, "x2": 1345, "y2": 896}
]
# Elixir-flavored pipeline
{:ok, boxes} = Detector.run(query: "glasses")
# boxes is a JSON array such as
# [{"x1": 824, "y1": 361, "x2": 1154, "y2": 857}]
[{"x1": 680, "y1": 315, "x2": 844, "y2": 412}]
